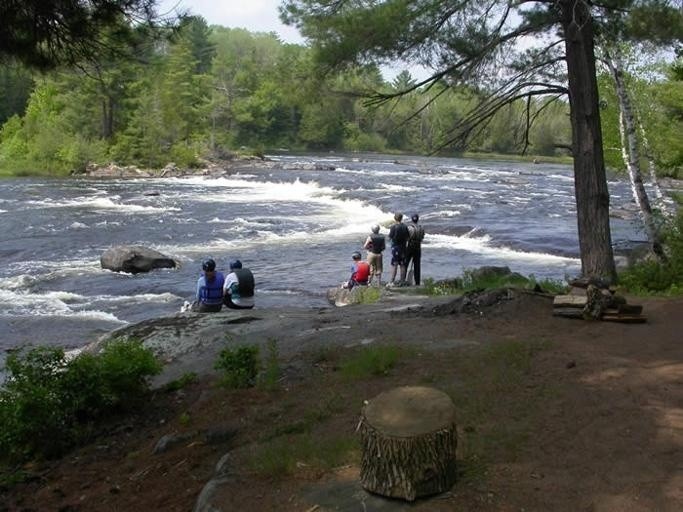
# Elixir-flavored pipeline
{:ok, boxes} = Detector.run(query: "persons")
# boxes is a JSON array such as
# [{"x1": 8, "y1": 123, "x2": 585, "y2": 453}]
[
  {"x1": 386, "y1": 212, "x2": 409, "y2": 288},
  {"x1": 181, "y1": 258, "x2": 225, "y2": 313},
  {"x1": 222, "y1": 260, "x2": 255, "y2": 310},
  {"x1": 363, "y1": 224, "x2": 385, "y2": 288},
  {"x1": 405, "y1": 213, "x2": 425, "y2": 286},
  {"x1": 341, "y1": 251, "x2": 371, "y2": 289}
]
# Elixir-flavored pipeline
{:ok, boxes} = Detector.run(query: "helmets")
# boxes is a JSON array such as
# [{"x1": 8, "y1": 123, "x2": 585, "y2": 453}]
[
  {"x1": 351, "y1": 251, "x2": 361, "y2": 260},
  {"x1": 203, "y1": 259, "x2": 215, "y2": 272},
  {"x1": 371, "y1": 223, "x2": 380, "y2": 233},
  {"x1": 230, "y1": 259, "x2": 242, "y2": 270}
]
